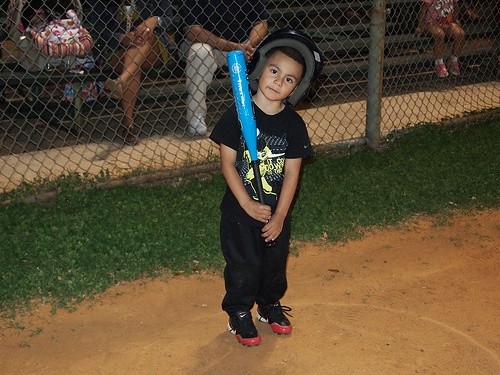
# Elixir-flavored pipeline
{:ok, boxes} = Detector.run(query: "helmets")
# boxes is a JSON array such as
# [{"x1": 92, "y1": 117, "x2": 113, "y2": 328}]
[{"x1": 246, "y1": 28, "x2": 323, "y2": 109}]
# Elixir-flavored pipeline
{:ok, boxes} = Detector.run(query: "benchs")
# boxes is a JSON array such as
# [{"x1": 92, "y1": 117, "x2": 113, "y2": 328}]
[{"x1": 0, "y1": 0, "x2": 500, "y2": 136}]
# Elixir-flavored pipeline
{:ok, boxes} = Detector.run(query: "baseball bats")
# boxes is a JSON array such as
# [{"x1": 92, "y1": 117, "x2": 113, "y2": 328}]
[{"x1": 227, "y1": 51, "x2": 276, "y2": 248}]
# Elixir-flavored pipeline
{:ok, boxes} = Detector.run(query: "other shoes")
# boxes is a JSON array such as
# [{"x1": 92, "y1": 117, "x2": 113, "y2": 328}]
[
  {"x1": 122, "y1": 126, "x2": 139, "y2": 146},
  {"x1": 103, "y1": 79, "x2": 123, "y2": 100}
]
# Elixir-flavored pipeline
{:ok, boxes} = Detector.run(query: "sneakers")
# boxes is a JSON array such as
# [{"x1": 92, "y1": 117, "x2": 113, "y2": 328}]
[
  {"x1": 228, "y1": 308, "x2": 261, "y2": 347},
  {"x1": 433, "y1": 62, "x2": 449, "y2": 78},
  {"x1": 446, "y1": 59, "x2": 462, "y2": 76},
  {"x1": 256, "y1": 301, "x2": 293, "y2": 336},
  {"x1": 188, "y1": 120, "x2": 208, "y2": 138}
]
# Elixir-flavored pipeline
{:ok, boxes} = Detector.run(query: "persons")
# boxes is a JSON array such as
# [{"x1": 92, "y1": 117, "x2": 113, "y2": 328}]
[
  {"x1": 11, "y1": 0, "x2": 268, "y2": 145},
  {"x1": 422, "y1": 0, "x2": 466, "y2": 78},
  {"x1": 209, "y1": 27, "x2": 325, "y2": 346}
]
